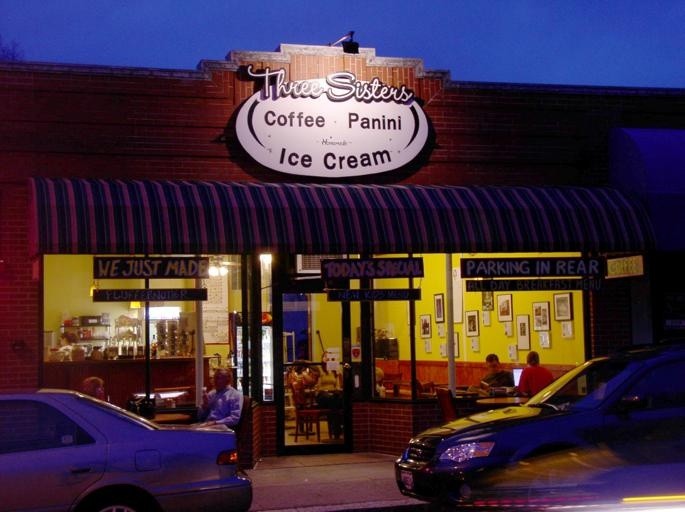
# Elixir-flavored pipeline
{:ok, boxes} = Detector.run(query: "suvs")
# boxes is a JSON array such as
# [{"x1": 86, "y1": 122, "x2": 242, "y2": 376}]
[{"x1": 391, "y1": 339, "x2": 684, "y2": 511}]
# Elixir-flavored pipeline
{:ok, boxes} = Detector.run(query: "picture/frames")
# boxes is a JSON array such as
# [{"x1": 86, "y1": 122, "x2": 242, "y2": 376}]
[{"x1": 419, "y1": 290, "x2": 573, "y2": 357}]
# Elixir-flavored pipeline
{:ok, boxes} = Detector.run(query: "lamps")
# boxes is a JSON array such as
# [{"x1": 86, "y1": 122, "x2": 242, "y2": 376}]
[
  {"x1": 130, "y1": 301, "x2": 141, "y2": 309},
  {"x1": 90, "y1": 280, "x2": 98, "y2": 296}
]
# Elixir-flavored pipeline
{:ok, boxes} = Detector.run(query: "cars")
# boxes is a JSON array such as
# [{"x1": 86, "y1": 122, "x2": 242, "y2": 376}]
[
  {"x1": 0, "y1": 387, "x2": 254, "y2": 511},
  {"x1": 451, "y1": 438, "x2": 684, "y2": 510}
]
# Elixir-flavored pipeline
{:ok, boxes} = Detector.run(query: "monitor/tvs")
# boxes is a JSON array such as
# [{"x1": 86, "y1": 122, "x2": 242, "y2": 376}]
[{"x1": 512, "y1": 367, "x2": 524, "y2": 387}]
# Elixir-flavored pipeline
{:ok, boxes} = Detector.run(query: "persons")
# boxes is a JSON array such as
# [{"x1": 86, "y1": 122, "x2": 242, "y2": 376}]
[
  {"x1": 479, "y1": 354, "x2": 515, "y2": 391},
  {"x1": 517, "y1": 350, "x2": 555, "y2": 395},
  {"x1": 315, "y1": 351, "x2": 342, "y2": 439},
  {"x1": 191, "y1": 368, "x2": 243, "y2": 428},
  {"x1": 82, "y1": 378, "x2": 108, "y2": 401},
  {"x1": 57, "y1": 331, "x2": 79, "y2": 361}
]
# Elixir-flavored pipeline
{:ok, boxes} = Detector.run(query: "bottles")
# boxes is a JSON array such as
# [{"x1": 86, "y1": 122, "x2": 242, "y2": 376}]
[{"x1": 103, "y1": 334, "x2": 159, "y2": 359}]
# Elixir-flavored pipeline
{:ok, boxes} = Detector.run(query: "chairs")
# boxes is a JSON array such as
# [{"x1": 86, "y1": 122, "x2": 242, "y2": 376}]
[
  {"x1": 434, "y1": 386, "x2": 458, "y2": 425},
  {"x1": 227, "y1": 395, "x2": 253, "y2": 479},
  {"x1": 288, "y1": 382, "x2": 332, "y2": 443}
]
